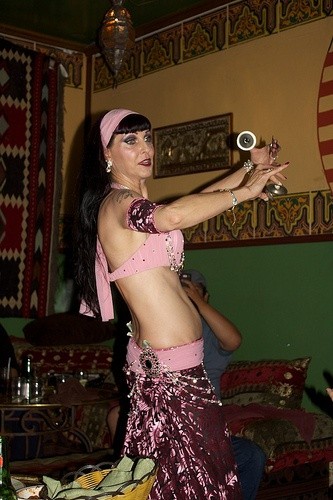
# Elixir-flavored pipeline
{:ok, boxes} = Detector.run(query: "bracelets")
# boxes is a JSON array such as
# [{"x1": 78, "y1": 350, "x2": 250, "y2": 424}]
[
  {"x1": 225, "y1": 189, "x2": 237, "y2": 212},
  {"x1": 243, "y1": 160, "x2": 253, "y2": 171}
]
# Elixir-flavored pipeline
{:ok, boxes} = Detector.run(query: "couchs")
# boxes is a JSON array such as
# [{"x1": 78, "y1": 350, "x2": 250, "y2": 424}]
[{"x1": 0, "y1": 337, "x2": 333, "y2": 500}]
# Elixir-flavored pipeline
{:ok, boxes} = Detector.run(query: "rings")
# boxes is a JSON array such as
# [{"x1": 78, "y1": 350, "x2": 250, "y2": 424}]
[
  {"x1": 274, "y1": 156, "x2": 278, "y2": 160},
  {"x1": 266, "y1": 168, "x2": 272, "y2": 171}
]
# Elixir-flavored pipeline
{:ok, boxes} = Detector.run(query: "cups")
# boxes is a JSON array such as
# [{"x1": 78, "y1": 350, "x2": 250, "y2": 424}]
[
  {"x1": 0, "y1": 367, "x2": 13, "y2": 396},
  {"x1": 0, "y1": 435, "x2": 9, "y2": 490}
]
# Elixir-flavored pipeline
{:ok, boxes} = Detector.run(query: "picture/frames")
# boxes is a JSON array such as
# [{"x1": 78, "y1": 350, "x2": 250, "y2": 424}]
[{"x1": 152, "y1": 113, "x2": 234, "y2": 180}]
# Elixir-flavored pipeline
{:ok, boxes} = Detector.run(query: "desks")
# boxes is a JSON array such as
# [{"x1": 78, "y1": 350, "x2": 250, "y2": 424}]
[{"x1": 0, "y1": 385, "x2": 119, "y2": 460}]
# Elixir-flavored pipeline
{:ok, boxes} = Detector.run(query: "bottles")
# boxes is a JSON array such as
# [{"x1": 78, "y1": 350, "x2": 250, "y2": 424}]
[
  {"x1": 21, "y1": 358, "x2": 32, "y2": 400},
  {"x1": 33, "y1": 376, "x2": 42, "y2": 397}
]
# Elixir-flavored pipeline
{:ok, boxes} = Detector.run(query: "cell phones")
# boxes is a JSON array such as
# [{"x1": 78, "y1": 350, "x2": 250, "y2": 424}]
[{"x1": 179, "y1": 272, "x2": 190, "y2": 289}]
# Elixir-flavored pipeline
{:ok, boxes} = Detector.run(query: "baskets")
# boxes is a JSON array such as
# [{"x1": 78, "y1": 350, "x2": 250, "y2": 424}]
[{"x1": 39, "y1": 455, "x2": 159, "y2": 500}]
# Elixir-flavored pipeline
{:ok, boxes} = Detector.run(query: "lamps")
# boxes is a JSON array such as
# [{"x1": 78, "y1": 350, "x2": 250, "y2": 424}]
[{"x1": 98, "y1": 0, "x2": 134, "y2": 89}]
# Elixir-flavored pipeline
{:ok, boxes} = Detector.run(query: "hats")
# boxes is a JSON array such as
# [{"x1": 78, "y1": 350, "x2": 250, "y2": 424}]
[{"x1": 181, "y1": 270, "x2": 206, "y2": 287}]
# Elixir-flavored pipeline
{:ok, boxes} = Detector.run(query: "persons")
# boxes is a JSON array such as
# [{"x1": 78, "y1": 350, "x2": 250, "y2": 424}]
[
  {"x1": 108, "y1": 270, "x2": 265, "y2": 500},
  {"x1": 0, "y1": 324, "x2": 39, "y2": 463},
  {"x1": 63, "y1": 109, "x2": 290, "y2": 500}
]
